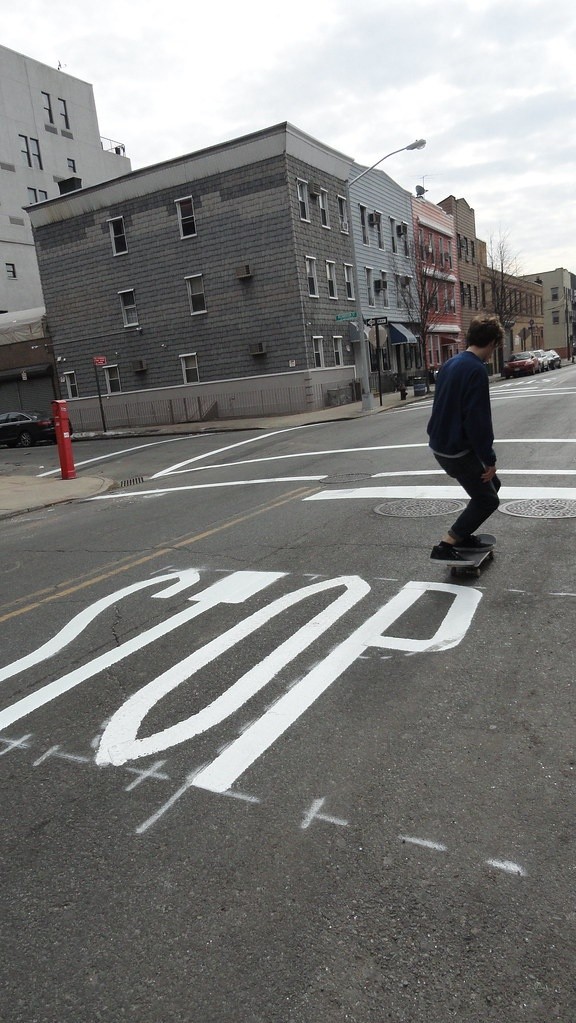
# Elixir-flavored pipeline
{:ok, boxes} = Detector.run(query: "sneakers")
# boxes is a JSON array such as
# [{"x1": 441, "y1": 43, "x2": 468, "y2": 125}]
[
  {"x1": 429, "y1": 545, "x2": 475, "y2": 565},
  {"x1": 453, "y1": 534, "x2": 495, "y2": 553}
]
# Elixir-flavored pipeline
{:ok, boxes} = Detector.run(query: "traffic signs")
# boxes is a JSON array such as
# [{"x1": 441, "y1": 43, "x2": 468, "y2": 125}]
[{"x1": 364, "y1": 317, "x2": 388, "y2": 327}]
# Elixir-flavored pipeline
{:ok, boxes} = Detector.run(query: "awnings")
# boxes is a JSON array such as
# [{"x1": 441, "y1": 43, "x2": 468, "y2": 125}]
[
  {"x1": 390, "y1": 323, "x2": 417, "y2": 345},
  {"x1": 349, "y1": 321, "x2": 372, "y2": 343}
]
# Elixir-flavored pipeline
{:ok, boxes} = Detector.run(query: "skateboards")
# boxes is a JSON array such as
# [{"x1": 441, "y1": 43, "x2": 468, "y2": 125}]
[{"x1": 446, "y1": 534, "x2": 497, "y2": 577}]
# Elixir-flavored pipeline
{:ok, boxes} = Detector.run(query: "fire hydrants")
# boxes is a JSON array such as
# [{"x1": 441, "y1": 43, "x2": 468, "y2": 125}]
[{"x1": 400, "y1": 387, "x2": 408, "y2": 400}]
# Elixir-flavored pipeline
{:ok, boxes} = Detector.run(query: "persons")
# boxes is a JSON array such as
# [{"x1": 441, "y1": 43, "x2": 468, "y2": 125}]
[{"x1": 426, "y1": 314, "x2": 505, "y2": 566}]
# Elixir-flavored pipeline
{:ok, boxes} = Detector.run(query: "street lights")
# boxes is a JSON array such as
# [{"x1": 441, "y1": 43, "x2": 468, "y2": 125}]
[{"x1": 344, "y1": 140, "x2": 427, "y2": 411}]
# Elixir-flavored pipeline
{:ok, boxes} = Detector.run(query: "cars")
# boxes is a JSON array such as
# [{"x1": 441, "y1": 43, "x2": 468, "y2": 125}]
[
  {"x1": 0, "y1": 411, "x2": 73, "y2": 447},
  {"x1": 501, "y1": 350, "x2": 561, "y2": 379}
]
